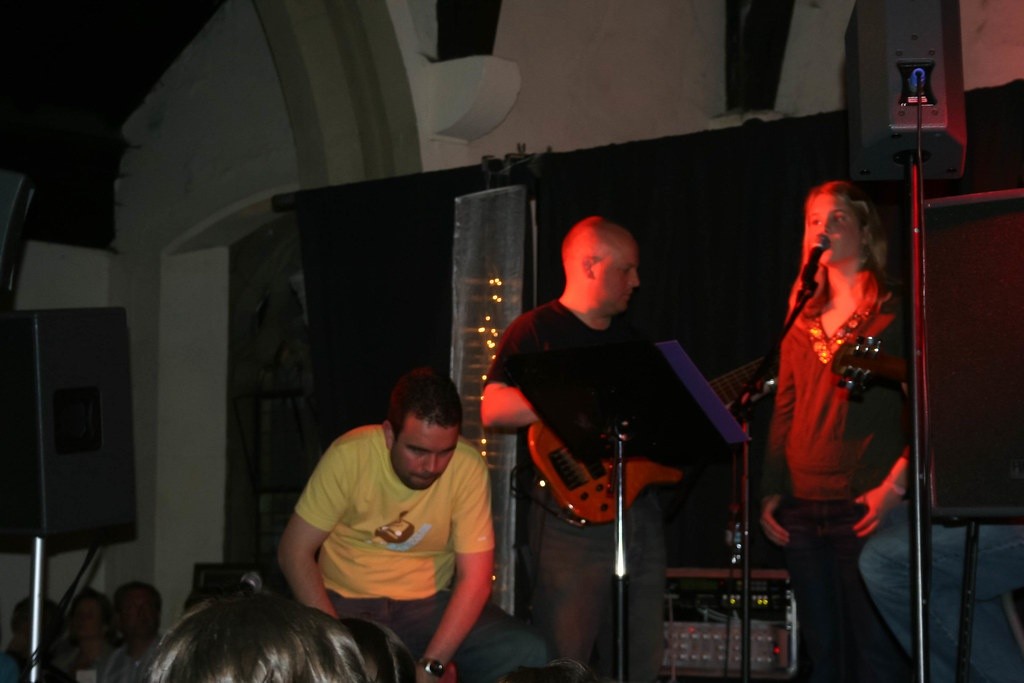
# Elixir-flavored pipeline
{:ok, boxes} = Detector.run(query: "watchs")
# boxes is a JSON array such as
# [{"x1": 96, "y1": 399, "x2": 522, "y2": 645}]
[{"x1": 417, "y1": 657, "x2": 445, "y2": 679}]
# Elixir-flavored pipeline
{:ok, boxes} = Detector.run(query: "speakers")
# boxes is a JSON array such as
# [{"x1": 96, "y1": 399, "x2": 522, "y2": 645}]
[
  {"x1": 844, "y1": 0, "x2": 966, "y2": 180},
  {"x1": 915, "y1": 188, "x2": 1023, "y2": 526},
  {"x1": 0, "y1": 306, "x2": 143, "y2": 556}
]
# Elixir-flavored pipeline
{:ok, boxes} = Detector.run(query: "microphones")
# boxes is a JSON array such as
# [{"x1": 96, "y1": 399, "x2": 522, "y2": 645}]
[
  {"x1": 238, "y1": 570, "x2": 262, "y2": 596},
  {"x1": 797, "y1": 234, "x2": 831, "y2": 302}
]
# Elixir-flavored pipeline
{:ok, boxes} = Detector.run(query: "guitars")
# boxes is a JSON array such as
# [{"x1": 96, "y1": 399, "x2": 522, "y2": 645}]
[
  {"x1": 525, "y1": 354, "x2": 778, "y2": 528},
  {"x1": 828, "y1": 334, "x2": 910, "y2": 396}
]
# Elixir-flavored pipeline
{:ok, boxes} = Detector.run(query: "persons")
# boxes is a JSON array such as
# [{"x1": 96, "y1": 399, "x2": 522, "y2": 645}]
[
  {"x1": 758, "y1": 180, "x2": 909, "y2": 682},
  {"x1": 38, "y1": 588, "x2": 140, "y2": 683},
  {"x1": 277, "y1": 366, "x2": 547, "y2": 683},
  {"x1": 96, "y1": 581, "x2": 163, "y2": 683},
  {"x1": 494, "y1": 656, "x2": 616, "y2": 683},
  {"x1": 479, "y1": 215, "x2": 708, "y2": 682},
  {"x1": 858, "y1": 499, "x2": 1024, "y2": 683},
  {"x1": 183, "y1": 584, "x2": 222, "y2": 616},
  {"x1": 0, "y1": 594, "x2": 79, "y2": 683},
  {"x1": 107, "y1": 592, "x2": 372, "y2": 683}
]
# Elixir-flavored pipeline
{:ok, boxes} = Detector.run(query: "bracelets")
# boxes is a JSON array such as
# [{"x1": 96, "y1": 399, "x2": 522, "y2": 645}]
[{"x1": 883, "y1": 479, "x2": 905, "y2": 497}]
